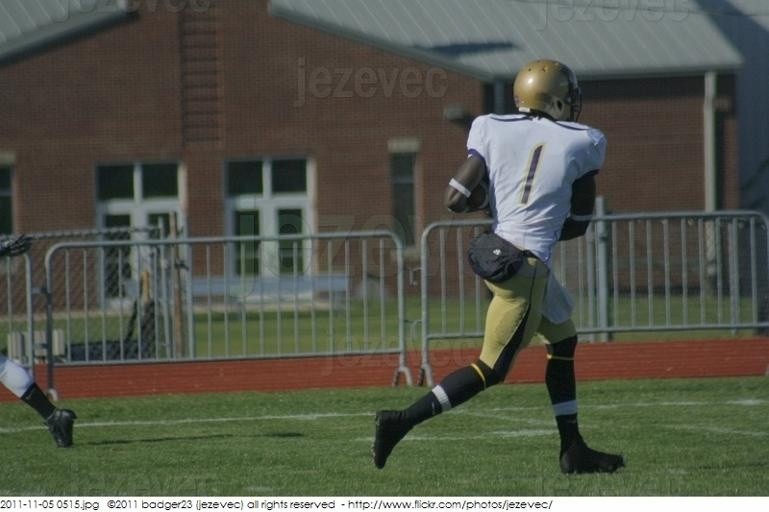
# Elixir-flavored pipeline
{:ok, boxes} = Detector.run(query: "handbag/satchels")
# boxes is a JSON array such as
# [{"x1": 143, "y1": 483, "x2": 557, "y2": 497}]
[{"x1": 466, "y1": 229, "x2": 524, "y2": 285}]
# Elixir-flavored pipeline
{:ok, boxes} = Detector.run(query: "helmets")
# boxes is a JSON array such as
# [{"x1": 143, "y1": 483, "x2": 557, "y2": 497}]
[{"x1": 512, "y1": 58, "x2": 583, "y2": 122}]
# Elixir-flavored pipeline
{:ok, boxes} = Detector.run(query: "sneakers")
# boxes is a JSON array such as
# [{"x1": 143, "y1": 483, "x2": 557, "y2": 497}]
[
  {"x1": 373, "y1": 408, "x2": 414, "y2": 470},
  {"x1": 558, "y1": 435, "x2": 625, "y2": 475},
  {"x1": 42, "y1": 406, "x2": 77, "y2": 449}
]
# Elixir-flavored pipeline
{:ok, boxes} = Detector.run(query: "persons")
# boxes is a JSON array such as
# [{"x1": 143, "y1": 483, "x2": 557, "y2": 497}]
[
  {"x1": 0, "y1": 351, "x2": 77, "y2": 449},
  {"x1": 373, "y1": 59, "x2": 625, "y2": 475}
]
